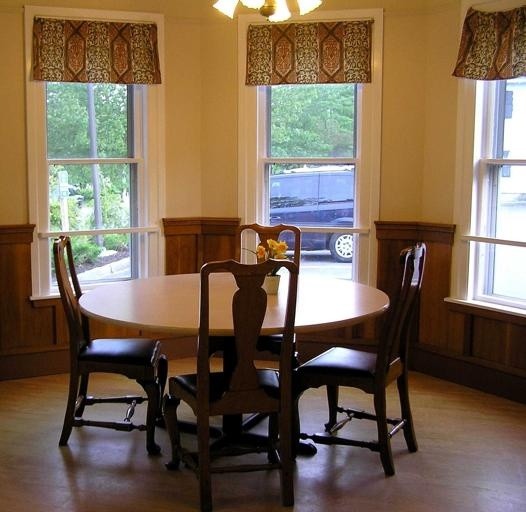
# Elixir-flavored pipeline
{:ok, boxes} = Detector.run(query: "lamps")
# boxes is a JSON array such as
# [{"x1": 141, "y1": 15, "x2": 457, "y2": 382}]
[{"x1": 211, "y1": 0, "x2": 324, "y2": 24}]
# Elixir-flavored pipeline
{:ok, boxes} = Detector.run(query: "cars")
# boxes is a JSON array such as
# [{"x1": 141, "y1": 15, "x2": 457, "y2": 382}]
[{"x1": 270, "y1": 168, "x2": 355, "y2": 262}]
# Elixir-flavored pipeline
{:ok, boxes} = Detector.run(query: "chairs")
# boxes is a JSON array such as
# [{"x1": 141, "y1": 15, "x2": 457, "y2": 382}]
[
  {"x1": 53, "y1": 234, "x2": 170, "y2": 459},
  {"x1": 288, "y1": 243, "x2": 425, "y2": 479},
  {"x1": 160, "y1": 257, "x2": 302, "y2": 512},
  {"x1": 234, "y1": 222, "x2": 304, "y2": 278}
]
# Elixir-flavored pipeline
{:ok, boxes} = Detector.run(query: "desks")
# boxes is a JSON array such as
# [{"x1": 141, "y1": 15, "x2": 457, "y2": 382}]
[{"x1": 79, "y1": 271, "x2": 395, "y2": 466}]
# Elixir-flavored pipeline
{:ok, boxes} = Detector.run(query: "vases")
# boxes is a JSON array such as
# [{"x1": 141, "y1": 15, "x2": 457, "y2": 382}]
[{"x1": 263, "y1": 274, "x2": 281, "y2": 296}]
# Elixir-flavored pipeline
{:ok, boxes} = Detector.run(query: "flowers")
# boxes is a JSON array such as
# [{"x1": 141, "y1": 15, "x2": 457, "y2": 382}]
[{"x1": 241, "y1": 238, "x2": 289, "y2": 273}]
